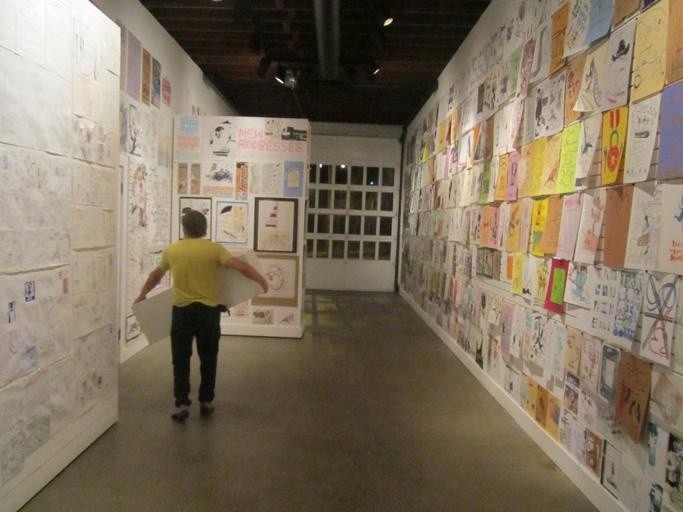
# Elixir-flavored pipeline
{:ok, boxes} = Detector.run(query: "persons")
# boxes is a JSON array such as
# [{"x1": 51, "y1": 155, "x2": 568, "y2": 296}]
[{"x1": 133, "y1": 210, "x2": 269, "y2": 420}]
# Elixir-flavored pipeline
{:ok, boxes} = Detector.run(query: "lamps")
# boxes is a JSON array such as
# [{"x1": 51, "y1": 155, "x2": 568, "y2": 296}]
[
  {"x1": 371, "y1": 2, "x2": 394, "y2": 27},
  {"x1": 273, "y1": 62, "x2": 287, "y2": 85}
]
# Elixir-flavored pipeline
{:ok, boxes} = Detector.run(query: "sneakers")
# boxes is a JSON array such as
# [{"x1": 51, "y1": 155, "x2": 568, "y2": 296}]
[
  {"x1": 200, "y1": 402, "x2": 217, "y2": 416},
  {"x1": 171, "y1": 404, "x2": 190, "y2": 420}
]
